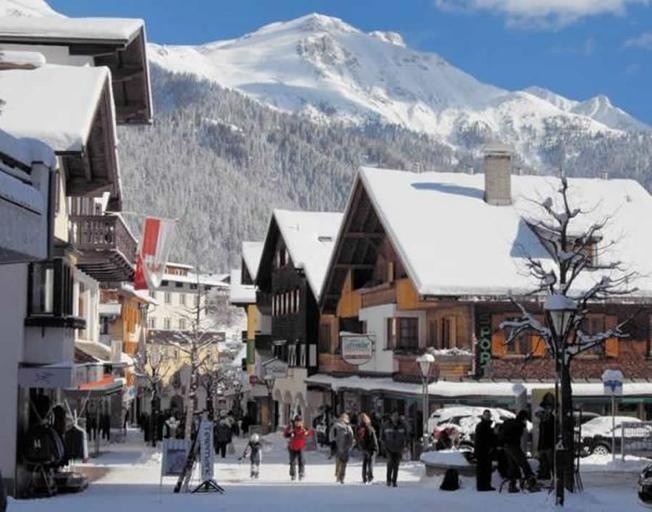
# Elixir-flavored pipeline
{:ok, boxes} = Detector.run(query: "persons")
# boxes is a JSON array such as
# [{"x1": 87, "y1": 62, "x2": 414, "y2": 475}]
[{"x1": 84, "y1": 400, "x2": 560, "y2": 493}]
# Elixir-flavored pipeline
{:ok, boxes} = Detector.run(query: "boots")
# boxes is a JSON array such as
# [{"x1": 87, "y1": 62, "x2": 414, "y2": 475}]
[
  {"x1": 508, "y1": 479, "x2": 519, "y2": 493},
  {"x1": 525, "y1": 473, "x2": 542, "y2": 493}
]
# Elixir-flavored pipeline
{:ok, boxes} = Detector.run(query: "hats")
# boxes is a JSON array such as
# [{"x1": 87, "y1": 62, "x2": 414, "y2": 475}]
[{"x1": 294, "y1": 415, "x2": 303, "y2": 422}]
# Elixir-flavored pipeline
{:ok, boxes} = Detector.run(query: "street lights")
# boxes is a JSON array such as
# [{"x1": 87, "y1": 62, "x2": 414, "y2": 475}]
[{"x1": 414, "y1": 353, "x2": 435, "y2": 452}]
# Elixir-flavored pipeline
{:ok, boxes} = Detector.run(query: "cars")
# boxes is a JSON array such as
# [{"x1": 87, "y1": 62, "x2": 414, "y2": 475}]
[
  {"x1": 422, "y1": 399, "x2": 652, "y2": 459},
  {"x1": 639, "y1": 459, "x2": 652, "y2": 507}
]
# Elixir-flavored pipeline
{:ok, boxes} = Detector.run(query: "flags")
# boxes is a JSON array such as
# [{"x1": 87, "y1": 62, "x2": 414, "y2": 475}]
[{"x1": 133, "y1": 215, "x2": 175, "y2": 292}]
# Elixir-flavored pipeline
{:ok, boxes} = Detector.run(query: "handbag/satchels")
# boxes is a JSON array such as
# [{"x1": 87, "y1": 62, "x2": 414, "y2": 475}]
[{"x1": 330, "y1": 439, "x2": 339, "y2": 454}]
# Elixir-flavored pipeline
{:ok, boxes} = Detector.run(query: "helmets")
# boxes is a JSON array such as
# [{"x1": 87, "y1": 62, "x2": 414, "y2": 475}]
[{"x1": 250, "y1": 434, "x2": 261, "y2": 442}]
